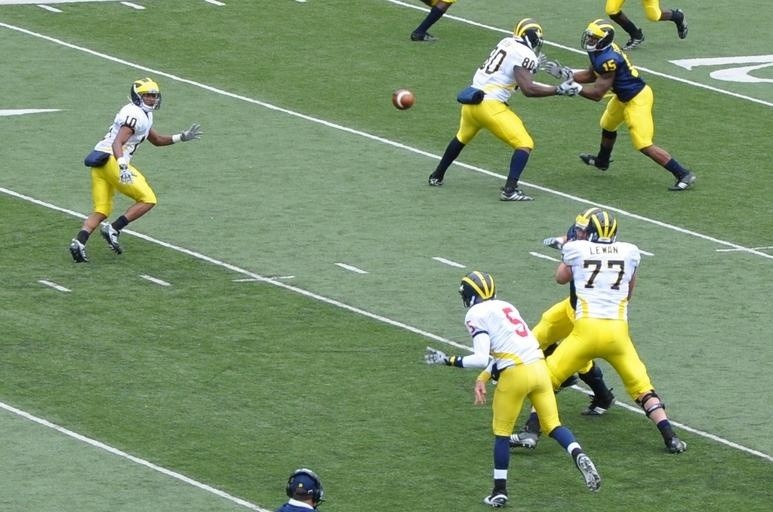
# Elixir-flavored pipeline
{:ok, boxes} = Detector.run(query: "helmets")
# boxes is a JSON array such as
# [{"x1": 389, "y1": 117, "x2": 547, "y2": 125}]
[
  {"x1": 286, "y1": 468, "x2": 323, "y2": 507},
  {"x1": 131, "y1": 77, "x2": 161, "y2": 112},
  {"x1": 514, "y1": 17, "x2": 543, "y2": 49},
  {"x1": 575, "y1": 207, "x2": 617, "y2": 243},
  {"x1": 580, "y1": 19, "x2": 615, "y2": 52},
  {"x1": 459, "y1": 270, "x2": 495, "y2": 308}
]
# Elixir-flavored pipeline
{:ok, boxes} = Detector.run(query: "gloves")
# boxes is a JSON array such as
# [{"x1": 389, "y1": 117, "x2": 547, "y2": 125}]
[
  {"x1": 119, "y1": 164, "x2": 136, "y2": 184},
  {"x1": 538, "y1": 54, "x2": 583, "y2": 96},
  {"x1": 543, "y1": 238, "x2": 563, "y2": 249},
  {"x1": 181, "y1": 124, "x2": 203, "y2": 141},
  {"x1": 425, "y1": 346, "x2": 450, "y2": 365}
]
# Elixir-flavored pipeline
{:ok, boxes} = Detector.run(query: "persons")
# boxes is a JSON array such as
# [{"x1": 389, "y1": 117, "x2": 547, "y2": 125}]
[
  {"x1": 508, "y1": 211, "x2": 688, "y2": 451},
  {"x1": 425, "y1": 17, "x2": 578, "y2": 201},
  {"x1": 531, "y1": 205, "x2": 614, "y2": 416},
  {"x1": 272, "y1": 467, "x2": 323, "y2": 511},
  {"x1": 68, "y1": 77, "x2": 203, "y2": 262},
  {"x1": 543, "y1": 18, "x2": 695, "y2": 190},
  {"x1": 423, "y1": 271, "x2": 600, "y2": 505},
  {"x1": 604, "y1": 0, "x2": 688, "y2": 49},
  {"x1": 406, "y1": 0, "x2": 452, "y2": 41}
]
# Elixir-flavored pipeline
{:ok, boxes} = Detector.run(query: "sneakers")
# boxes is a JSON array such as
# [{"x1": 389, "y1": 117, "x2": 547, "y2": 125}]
[
  {"x1": 70, "y1": 224, "x2": 122, "y2": 263},
  {"x1": 668, "y1": 172, "x2": 695, "y2": 191},
  {"x1": 621, "y1": 8, "x2": 687, "y2": 50},
  {"x1": 579, "y1": 152, "x2": 609, "y2": 170},
  {"x1": 668, "y1": 437, "x2": 687, "y2": 452},
  {"x1": 576, "y1": 453, "x2": 600, "y2": 491},
  {"x1": 429, "y1": 174, "x2": 443, "y2": 185},
  {"x1": 581, "y1": 392, "x2": 615, "y2": 415},
  {"x1": 411, "y1": 31, "x2": 438, "y2": 41},
  {"x1": 500, "y1": 188, "x2": 533, "y2": 200},
  {"x1": 484, "y1": 491, "x2": 507, "y2": 507},
  {"x1": 509, "y1": 432, "x2": 538, "y2": 449}
]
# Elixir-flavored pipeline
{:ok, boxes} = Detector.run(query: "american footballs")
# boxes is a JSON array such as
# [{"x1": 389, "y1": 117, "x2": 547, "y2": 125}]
[{"x1": 392, "y1": 88, "x2": 415, "y2": 110}]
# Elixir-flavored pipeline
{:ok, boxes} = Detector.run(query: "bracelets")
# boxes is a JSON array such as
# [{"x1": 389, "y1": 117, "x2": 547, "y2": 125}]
[
  {"x1": 117, "y1": 157, "x2": 127, "y2": 165},
  {"x1": 171, "y1": 134, "x2": 181, "y2": 144}
]
